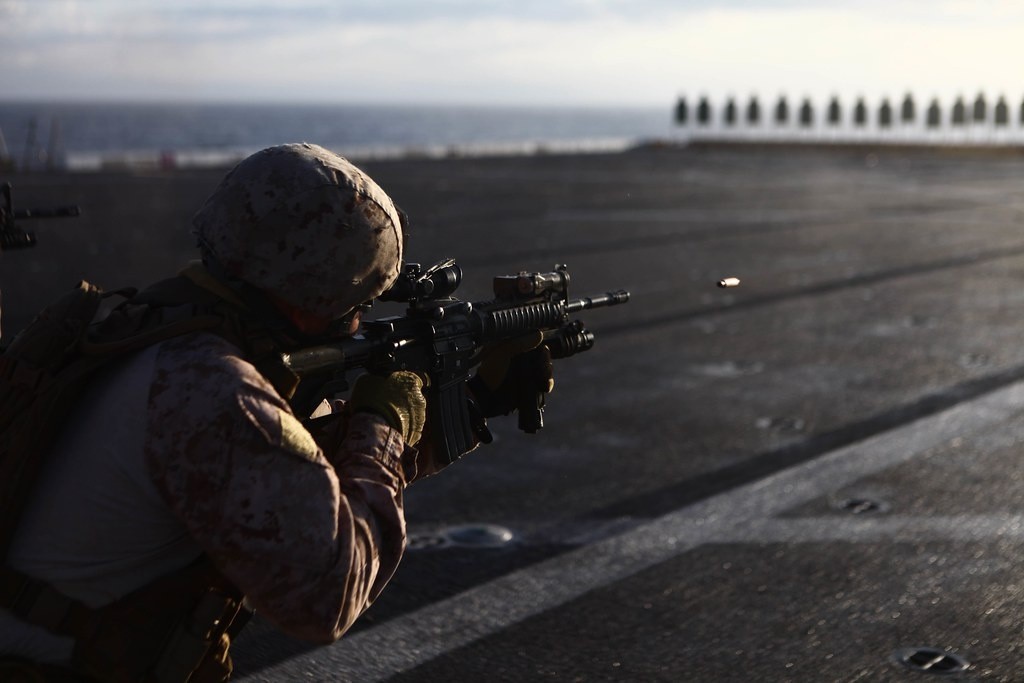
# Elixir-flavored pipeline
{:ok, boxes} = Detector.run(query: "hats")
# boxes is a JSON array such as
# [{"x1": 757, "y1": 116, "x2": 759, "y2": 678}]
[{"x1": 194, "y1": 138, "x2": 405, "y2": 327}]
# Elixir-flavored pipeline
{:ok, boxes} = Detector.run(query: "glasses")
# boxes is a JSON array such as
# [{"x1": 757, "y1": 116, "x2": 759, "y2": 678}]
[{"x1": 326, "y1": 296, "x2": 373, "y2": 342}]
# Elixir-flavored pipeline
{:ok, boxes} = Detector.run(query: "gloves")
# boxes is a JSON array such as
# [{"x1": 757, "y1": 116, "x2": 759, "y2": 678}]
[{"x1": 356, "y1": 368, "x2": 428, "y2": 450}]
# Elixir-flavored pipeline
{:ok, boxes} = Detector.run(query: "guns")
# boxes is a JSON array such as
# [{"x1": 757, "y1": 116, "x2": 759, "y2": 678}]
[{"x1": 260, "y1": 263, "x2": 629, "y2": 466}]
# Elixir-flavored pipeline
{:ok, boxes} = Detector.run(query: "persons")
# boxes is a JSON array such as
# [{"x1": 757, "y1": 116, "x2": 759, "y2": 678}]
[{"x1": 1, "y1": 142, "x2": 559, "y2": 682}]
